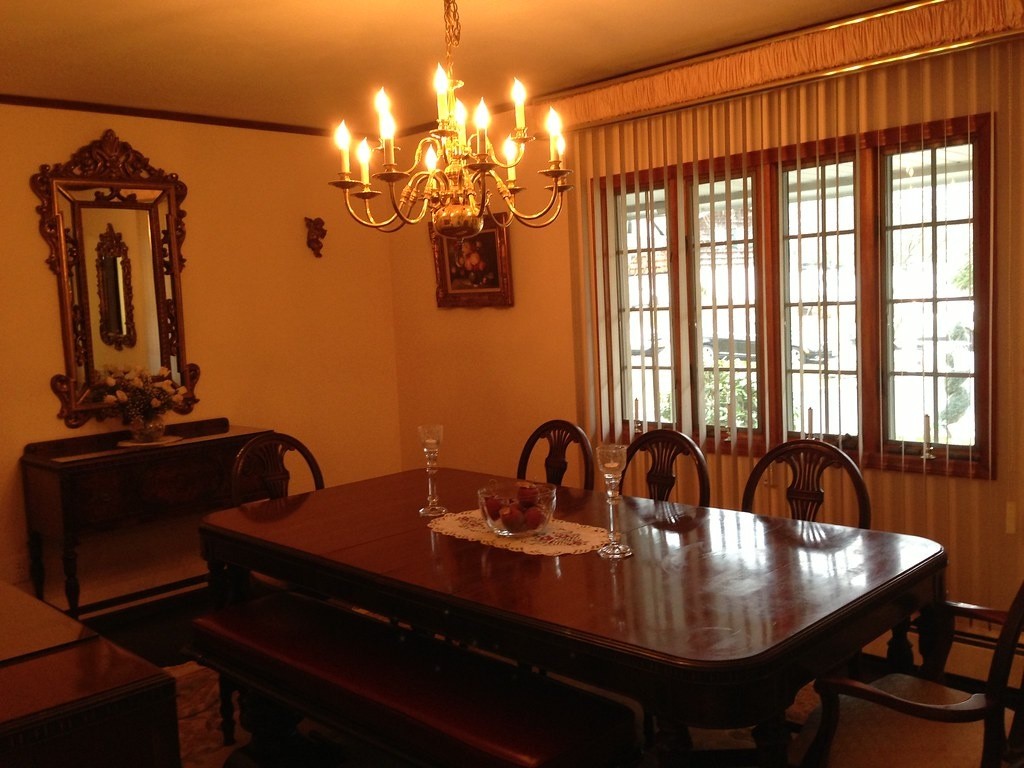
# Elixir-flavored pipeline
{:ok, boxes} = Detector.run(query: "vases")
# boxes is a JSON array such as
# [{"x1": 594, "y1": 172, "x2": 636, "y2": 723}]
[{"x1": 130, "y1": 412, "x2": 166, "y2": 440}]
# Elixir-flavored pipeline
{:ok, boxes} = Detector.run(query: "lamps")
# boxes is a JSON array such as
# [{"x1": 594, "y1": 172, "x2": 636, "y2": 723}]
[{"x1": 331, "y1": 0, "x2": 573, "y2": 261}]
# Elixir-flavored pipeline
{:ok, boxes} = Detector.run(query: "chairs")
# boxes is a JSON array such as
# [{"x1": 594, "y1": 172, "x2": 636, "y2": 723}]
[
  {"x1": 517, "y1": 419, "x2": 596, "y2": 492},
  {"x1": 620, "y1": 429, "x2": 713, "y2": 506},
  {"x1": 741, "y1": 440, "x2": 873, "y2": 528},
  {"x1": 784, "y1": 578, "x2": 1024, "y2": 768}
]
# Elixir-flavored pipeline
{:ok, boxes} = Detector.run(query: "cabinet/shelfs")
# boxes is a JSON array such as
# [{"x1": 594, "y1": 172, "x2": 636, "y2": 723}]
[
  {"x1": 0, "y1": 579, "x2": 183, "y2": 767},
  {"x1": 20, "y1": 417, "x2": 273, "y2": 622}
]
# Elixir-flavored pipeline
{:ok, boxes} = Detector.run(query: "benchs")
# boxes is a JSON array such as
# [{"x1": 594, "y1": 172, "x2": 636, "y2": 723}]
[{"x1": 179, "y1": 589, "x2": 636, "y2": 768}]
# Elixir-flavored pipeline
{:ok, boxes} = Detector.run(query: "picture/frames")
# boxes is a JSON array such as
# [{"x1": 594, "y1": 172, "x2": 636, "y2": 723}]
[{"x1": 427, "y1": 212, "x2": 514, "y2": 310}]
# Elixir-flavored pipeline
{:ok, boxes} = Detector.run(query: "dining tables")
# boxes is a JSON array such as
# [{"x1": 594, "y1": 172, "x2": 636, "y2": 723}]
[{"x1": 198, "y1": 462, "x2": 954, "y2": 768}]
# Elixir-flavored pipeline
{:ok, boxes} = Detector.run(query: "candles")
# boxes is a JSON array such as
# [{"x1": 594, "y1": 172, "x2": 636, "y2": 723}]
[
  {"x1": 374, "y1": 86, "x2": 396, "y2": 164},
  {"x1": 356, "y1": 135, "x2": 373, "y2": 191},
  {"x1": 502, "y1": 133, "x2": 519, "y2": 180},
  {"x1": 455, "y1": 97, "x2": 468, "y2": 145},
  {"x1": 425, "y1": 143, "x2": 437, "y2": 187},
  {"x1": 509, "y1": 77, "x2": 528, "y2": 129},
  {"x1": 333, "y1": 119, "x2": 352, "y2": 180},
  {"x1": 474, "y1": 96, "x2": 491, "y2": 154},
  {"x1": 431, "y1": 61, "x2": 450, "y2": 122}
]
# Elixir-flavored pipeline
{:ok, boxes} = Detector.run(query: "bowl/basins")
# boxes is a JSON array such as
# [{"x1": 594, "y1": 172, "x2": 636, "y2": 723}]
[{"x1": 477, "y1": 480, "x2": 557, "y2": 539}]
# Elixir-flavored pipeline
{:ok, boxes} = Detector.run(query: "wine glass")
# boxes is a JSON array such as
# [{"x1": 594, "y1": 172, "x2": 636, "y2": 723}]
[
  {"x1": 417, "y1": 424, "x2": 448, "y2": 516},
  {"x1": 596, "y1": 445, "x2": 634, "y2": 559}
]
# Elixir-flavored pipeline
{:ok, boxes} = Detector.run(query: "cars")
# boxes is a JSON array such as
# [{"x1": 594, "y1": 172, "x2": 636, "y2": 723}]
[{"x1": 699, "y1": 333, "x2": 833, "y2": 373}]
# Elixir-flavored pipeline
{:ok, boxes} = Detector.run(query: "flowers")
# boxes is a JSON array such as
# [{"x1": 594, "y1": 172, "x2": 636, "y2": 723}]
[{"x1": 91, "y1": 364, "x2": 187, "y2": 420}]
